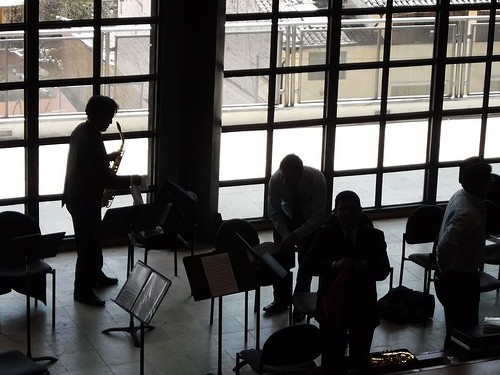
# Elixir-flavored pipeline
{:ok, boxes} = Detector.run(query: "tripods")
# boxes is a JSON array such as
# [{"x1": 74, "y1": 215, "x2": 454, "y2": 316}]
[
  {"x1": 0, "y1": 231, "x2": 66, "y2": 364},
  {"x1": 98, "y1": 200, "x2": 172, "y2": 348}
]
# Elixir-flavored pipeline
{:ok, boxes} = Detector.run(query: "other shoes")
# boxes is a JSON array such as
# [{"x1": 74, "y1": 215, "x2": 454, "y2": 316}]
[
  {"x1": 263, "y1": 300, "x2": 290, "y2": 313},
  {"x1": 91, "y1": 269, "x2": 118, "y2": 288},
  {"x1": 293, "y1": 310, "x2": 306, "y2": 322},
  {"x1": 74, "y1": 287, "x2": 105, "y2": 306}
]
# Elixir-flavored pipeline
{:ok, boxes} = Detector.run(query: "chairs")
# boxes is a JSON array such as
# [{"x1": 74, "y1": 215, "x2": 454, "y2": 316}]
[
  {"x1": 0, "y1": 350, "x2": 49, "y2": 375},
  {"x1": 210, "y1": 219, "x2": 260, "y2": 324},
  {"x1": 400, "y1": 206, "x2": 444, "y2": 293},
  {"x1": 480, "y1": 272, "x2": 499, "y2": 292},
  {"x1": 294, "y1": 292, "x2": 317, "y2": 325},
  {"x1": 0, "y1": 211, "x2": 55, "y2": 331}
]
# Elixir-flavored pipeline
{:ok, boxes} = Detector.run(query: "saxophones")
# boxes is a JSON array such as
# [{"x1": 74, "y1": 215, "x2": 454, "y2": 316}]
[
  {"x1": 101, "y1": 121, "x2": 127, "y2": 208},
  {"x1": 370, "y1": 346, "x2": 414, "y2": 366}
]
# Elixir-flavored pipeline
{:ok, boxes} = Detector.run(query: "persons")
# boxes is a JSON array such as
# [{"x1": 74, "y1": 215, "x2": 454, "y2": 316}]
[
  {"x1": 432, "y1": 156, "x2": 492, "y2": 364},
  {"x1": 61, "y1": 95, "x2": 123, "y2": 306},
  {"x1": 263, "y1": 154, "x2": 326, "y2": 322},
  {"x1": 309, "y1": 190, "x2": 390, "y2": 375}
]
